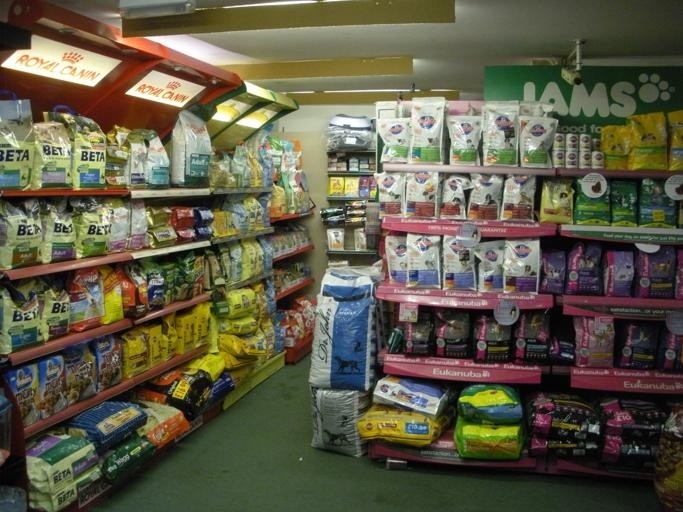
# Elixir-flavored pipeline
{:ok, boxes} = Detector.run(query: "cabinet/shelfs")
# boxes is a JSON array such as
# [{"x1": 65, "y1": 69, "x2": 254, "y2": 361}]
[
  {"x1": 324, "y1": 148, "x2": 381, "y2": 256},
  {"x1": 368, "y1": 163, "x2": 556, "y2": 472},
  {"x1": 211, "y1": 186, "x2": 287, "y2": 411},
  {"x1": 270, "y1": 212, "x2": 314, "y2": 363},
  {"x1": 548, "y1": 169, "x2": 683, "y2": 480},
  {"x1": 0, "y1": 188, "x2": 218, "y2": 511}
]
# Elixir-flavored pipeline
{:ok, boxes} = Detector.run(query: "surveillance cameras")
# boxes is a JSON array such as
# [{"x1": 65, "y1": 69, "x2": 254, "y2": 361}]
[
  {"x1": 559, "y1": 65, "x2": 584, "y2": 88},
  {"x1": 397, "y1": 92, "x2": 404, "y2": 102},
  {"x1": 409, "y1": 82, "x2": 418, "y2": 93}
]
